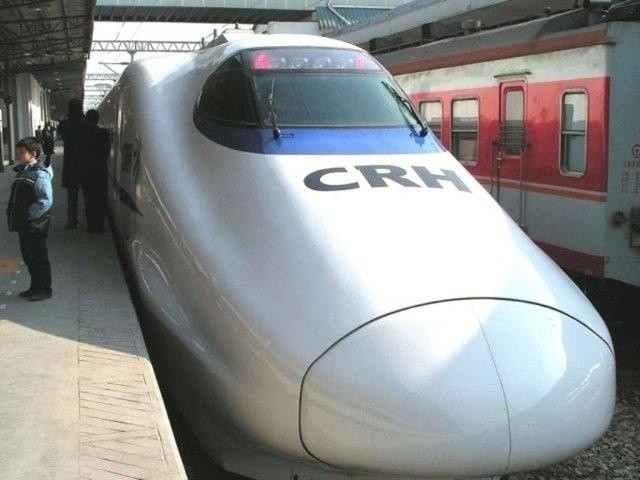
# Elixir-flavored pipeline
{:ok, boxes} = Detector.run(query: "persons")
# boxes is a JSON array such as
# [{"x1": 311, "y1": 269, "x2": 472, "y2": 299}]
[
  {"x1": 4, "y1": 134, "x2": 56, "y2": 304},
  {"x1": 35, "y1": 97, "x2": 112, "y2": 236}
]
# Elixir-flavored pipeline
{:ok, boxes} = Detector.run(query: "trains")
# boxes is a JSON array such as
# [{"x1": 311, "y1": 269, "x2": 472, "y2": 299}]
[
  {"x1": 94, "y1": 29, "x2": 622, "y2": 478},
  {"x1": 318, "y1": 0, "x2": 640, "y2": 303}
]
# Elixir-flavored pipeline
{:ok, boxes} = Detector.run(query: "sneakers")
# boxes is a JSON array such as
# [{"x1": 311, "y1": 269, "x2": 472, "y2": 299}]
[{"x1": 18, "y1": 285, "x2": 53, "y2": 302}]
